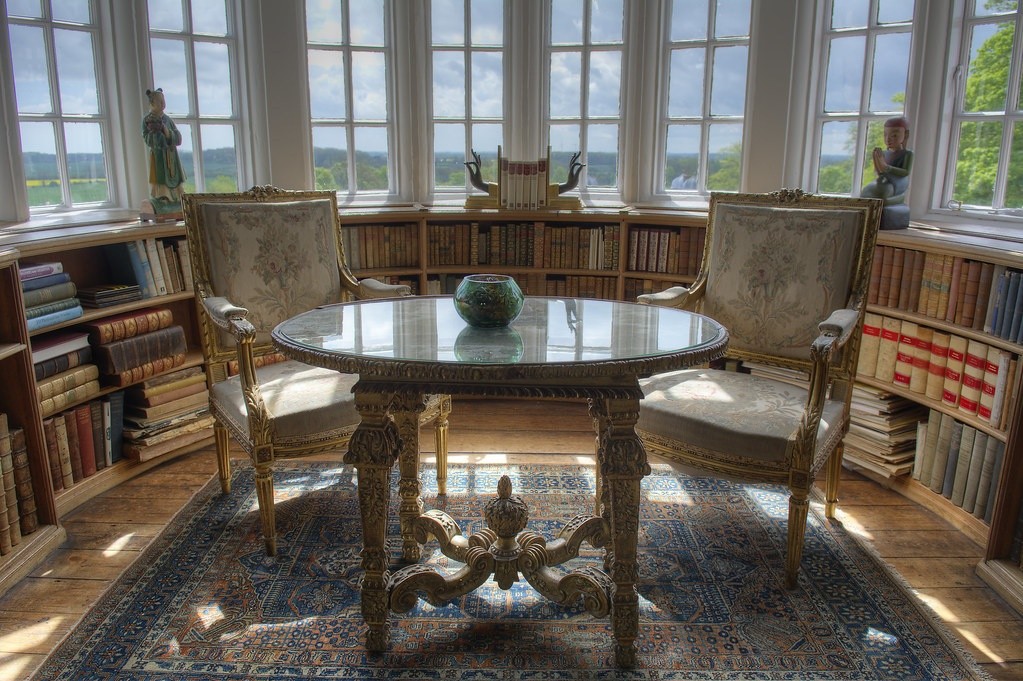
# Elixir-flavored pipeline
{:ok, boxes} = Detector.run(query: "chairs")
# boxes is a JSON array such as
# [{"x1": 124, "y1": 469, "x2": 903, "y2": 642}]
[
  {"x1": 595, "y1": 186, "x2": 886, "y2": 583},
  {"x1": 179, "y1": 183, "x2": 453, "y2": 561}
]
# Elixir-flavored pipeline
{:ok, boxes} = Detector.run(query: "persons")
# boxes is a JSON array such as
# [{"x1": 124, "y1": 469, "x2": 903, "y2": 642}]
[
  {"x1": 862, "y1": 117, "x2": 912, "y2": 204},
  {"x1": 143, "y1": 88, "x2": 187, "y2": 201},
  {"x1": 463, "y1": 149, "x2": 497, "y2": 198},
  {"x1": 550, "y1": 151, "x2": 586, "y2": 198},
  {"x1": 672, "y1": 158, "x2": 697, "y2": 189}
]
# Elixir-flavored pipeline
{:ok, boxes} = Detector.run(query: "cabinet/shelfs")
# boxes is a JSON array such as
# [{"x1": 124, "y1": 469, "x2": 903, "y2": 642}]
[
  {"x1": 338, "y1": 208, "x2": 708, "y2": 302},
  {"x1": 0, "y1": 221, "x2": 215, "y2": 596},
  {"x1": 841, "y1": 223, "x2": 1023, "y2": 554}
]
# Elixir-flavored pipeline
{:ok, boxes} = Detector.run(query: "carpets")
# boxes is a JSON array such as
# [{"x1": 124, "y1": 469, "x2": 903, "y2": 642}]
[{"x1": 27, "y1": 456, "x2": 1001, "y2": 681}]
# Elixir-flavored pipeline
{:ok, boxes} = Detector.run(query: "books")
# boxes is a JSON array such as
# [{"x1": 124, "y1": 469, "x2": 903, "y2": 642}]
[
  {"x1": 342, "y1": 222, "x2": 707, "y2": 302},
  {"x1": 741, "y1": 246, "x2": 1023, "y2": 526},
  {"x1": 499, "y1": 158, "x2": 547, "y2": 210},
  {"x1": 0, "y1": 236, "x2": 216, "y2": 556}
]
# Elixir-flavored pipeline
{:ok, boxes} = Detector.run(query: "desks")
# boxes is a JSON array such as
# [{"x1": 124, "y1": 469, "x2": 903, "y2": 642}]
[{"x1": 272, "y1": 292, "x2": 730, "y2": 671}]
